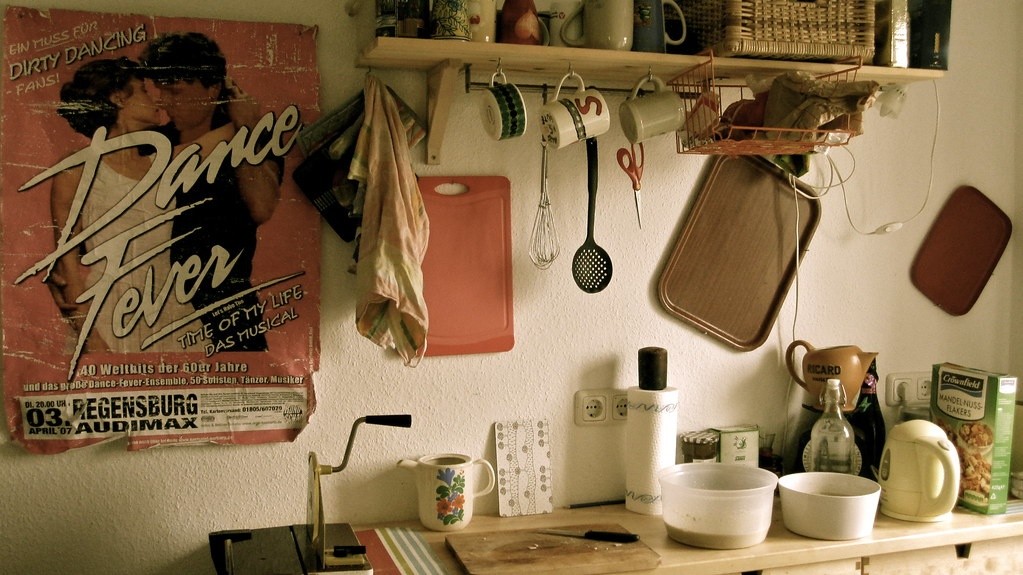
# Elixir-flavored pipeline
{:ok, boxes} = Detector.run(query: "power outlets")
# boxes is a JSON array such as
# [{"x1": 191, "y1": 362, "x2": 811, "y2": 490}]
[
  {"x1": 574, "y1": 390, "x2": 627, "y2": 425},
  {"x1": 886, "y1": 372, "x2": 932, "y2": 407}
]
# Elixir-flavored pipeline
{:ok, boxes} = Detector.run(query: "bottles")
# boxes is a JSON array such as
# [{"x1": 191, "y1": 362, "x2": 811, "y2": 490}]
[
  {"x1": 813, "y1": 378, "x2": 854, "y2": 474},
  {"x1": 850, "y1": 358, "x2": 886, "y2": 483}
]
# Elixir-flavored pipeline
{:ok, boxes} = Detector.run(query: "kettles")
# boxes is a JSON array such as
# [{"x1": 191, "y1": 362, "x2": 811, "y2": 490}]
[{"x1": 878, "y1": 418, "x2": 961, "y2": 523}]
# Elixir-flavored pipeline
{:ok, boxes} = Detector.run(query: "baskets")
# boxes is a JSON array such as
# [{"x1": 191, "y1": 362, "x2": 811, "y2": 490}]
[{"x1": 666, "y1": 0, "x2": 875, "y2": 63}]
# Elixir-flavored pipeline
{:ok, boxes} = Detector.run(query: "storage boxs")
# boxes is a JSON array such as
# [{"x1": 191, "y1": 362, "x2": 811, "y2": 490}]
[{"x1": 930, "y1": 362, "x2": 1017, "y2": 516}]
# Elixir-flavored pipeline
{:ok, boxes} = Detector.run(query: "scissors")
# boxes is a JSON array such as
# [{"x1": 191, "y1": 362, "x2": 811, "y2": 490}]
[{"x1": 617, "y1": 141, "x2": 644, "y2": 230}]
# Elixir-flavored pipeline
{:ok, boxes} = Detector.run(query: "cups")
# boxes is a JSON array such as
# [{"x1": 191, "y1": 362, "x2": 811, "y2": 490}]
[
  {"x1": 550, "y1": 0, "x2": 687, "y2": 54},
  {"x1": 539, "y1": 73, "x2": 610, "y2": 148},
  {"x1": 481, "y1": 71, "x2": 526, "y2": 142},
  {"x1": 620, "y1": 75, "x2": 686, "y2": 143}
]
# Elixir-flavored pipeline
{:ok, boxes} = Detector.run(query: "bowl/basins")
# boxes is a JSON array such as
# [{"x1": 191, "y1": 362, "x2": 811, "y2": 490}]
[
  {"x1": 956, "y1": 423, "x2": 993, "y2": 456},
  {"x1": 779, "y1": 471, "x2": 882, "y2": 539},
  {"x1": 657, "y1": 463, "x2": 779, "y2": 551}
]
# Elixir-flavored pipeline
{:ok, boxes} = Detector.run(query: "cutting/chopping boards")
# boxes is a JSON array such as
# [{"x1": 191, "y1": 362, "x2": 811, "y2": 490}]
[{"x1": 445, "y1": 523, "x2": 661, "y2": 574}]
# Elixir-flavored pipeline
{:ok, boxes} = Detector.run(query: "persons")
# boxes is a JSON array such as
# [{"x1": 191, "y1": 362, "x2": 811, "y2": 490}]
[
  {"x1": 44, "y1": 34, "x2": 284, "y2": 353},
  {"x1": 52, "y1": 55, "x2": 263, "y2": 353}
]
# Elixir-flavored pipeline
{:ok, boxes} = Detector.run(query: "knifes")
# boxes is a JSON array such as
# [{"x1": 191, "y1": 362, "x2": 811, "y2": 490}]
[{"x1": 534, "y1": 529, "x2": 641, "y2": 544}]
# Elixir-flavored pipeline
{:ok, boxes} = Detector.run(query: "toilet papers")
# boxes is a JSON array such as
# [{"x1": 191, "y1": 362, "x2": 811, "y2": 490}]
[{"x1": 623, "y1": 388, "x2": 678, "y2": 517}]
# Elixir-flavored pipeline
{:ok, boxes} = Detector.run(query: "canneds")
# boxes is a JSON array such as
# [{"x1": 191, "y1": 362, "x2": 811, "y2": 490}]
[{"x1": 679, "y1": 431, "x2": 719, "y2": 462}]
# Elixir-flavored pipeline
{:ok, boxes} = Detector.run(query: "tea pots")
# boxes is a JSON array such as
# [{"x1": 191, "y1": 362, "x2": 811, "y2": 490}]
[
  {"x1": 397, "y1": 454, "x2": 495, "y2": 532},
  {"x1": 786, "y1": 337, "x2": 880, "y2": 409}
]
